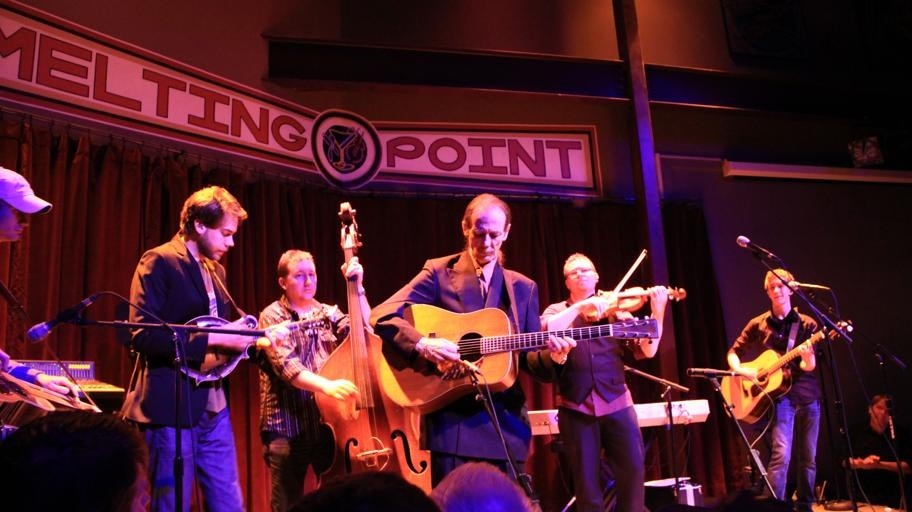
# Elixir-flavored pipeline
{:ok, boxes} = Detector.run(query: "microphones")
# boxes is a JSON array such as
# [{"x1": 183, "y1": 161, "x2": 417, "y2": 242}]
[
  {"x1": 625, "y1": 364, "x2": 689, "y2": 393},
  {"x1": 682, "y1": 366, "x2": 761, "y2": 389},
  {"x1": 26, "y1": 295, "x2": 94, "y2": 340},
  {"x1": 432, "y1": 348, "x2": 485, "y2": 380},
  {"x1": 737, "y1": 233, "x2": 780, "y2": 264}
]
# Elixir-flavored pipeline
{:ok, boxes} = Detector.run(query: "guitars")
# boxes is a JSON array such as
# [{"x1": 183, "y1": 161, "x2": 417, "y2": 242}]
[
  {"x1": 0, "y1": 371, "x2": 102, "y2": 426},
  {"x1": 379, "y1": 303, "x2": 659, "y2": 415},
  {"x1": 720, "y1": 320, "x2": 854, "y2": 424},
  {"x1": 173, "y1": 307, "x2": 332, "y2": 385}
]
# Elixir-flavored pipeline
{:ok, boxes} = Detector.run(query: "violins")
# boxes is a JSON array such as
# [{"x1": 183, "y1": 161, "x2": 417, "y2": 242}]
[{"x1": 583, "y1": 284, "x2": 687, "y2": 322}]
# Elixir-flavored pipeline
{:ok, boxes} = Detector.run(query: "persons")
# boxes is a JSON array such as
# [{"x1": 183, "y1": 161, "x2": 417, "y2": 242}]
[
  {"x1": 539, "y1": 252, "x2": 668, "y2": 512},
  {"x1": 429, "y1": 462, "x2": 533, "y2": 512},
  {"x1": 0, "y1": 409, "x2": 151, "y2": 512},
  {"x1": 368, "y1": 193, "x2": 580, "y2": 490},
  {"x1": 829, "y1": 395, "x2": 912, "y2": 509},
  {"x1": 255, "y1": 250, "x2": 373, "y2": 512},
  {"x1": 726, "y1": 269, "x2": 821, "y2": 512},
  {"x1": 121, "y1": 185, "x2": 293, "y2": 512},
  {"x1": 285, "y1": 470, "x2": 441, "y2": 512},
  {"x1": 1, "y1": 167, "x2": 80, "y2": 398}
]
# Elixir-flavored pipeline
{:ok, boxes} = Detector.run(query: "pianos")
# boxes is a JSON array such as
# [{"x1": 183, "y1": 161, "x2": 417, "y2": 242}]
[
  {"x1": 526, "y1": 400, "x2": 711, "y2": 435},
  {"x1": 1, "y1": 358, "x2": 126, "y2": 394}
]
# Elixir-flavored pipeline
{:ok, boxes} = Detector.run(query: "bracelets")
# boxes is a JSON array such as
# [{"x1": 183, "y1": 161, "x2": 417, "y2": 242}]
[
  {"x1": 358, "y1": 289, "x2": 366, "y2": 296},
  {"x1": 551, "y1": 353, "x2": 567, "y2": 367}
]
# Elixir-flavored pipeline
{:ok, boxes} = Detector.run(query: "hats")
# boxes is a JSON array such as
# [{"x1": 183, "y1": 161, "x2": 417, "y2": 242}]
[{"x1": 0, "y1": 166, "x2": 53, "y2": 215}]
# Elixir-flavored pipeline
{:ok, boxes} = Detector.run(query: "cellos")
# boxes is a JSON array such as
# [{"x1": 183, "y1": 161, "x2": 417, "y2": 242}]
[{"x1": 314, "y1": 200, "x2": 435, "y2": 497}]
